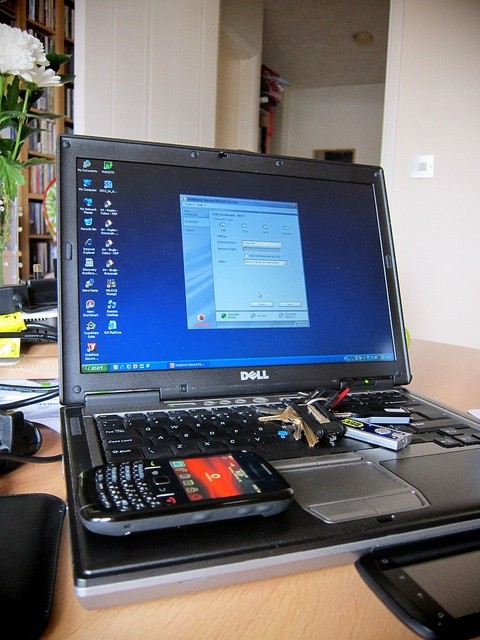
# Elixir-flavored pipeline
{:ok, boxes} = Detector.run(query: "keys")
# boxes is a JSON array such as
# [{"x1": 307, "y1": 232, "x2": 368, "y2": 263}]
[{"x1": 255, "y1": 386, "x2": 350, "y2": 450}]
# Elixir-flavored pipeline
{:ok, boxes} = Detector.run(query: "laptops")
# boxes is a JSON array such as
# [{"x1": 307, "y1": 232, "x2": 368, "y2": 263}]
[{"x1": 52, "y1": 133, "x2": 479, "y2": 615}]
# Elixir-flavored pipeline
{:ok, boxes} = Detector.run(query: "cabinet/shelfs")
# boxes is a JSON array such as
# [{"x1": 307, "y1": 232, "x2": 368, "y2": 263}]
[{"x1": 0, "y1": 9, "x2": 75, "y2": 286}]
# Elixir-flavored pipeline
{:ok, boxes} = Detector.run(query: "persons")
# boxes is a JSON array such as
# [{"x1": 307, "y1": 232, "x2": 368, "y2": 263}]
[{"x1": 25, "y1": 29, "x2": 54, "y2": 56}]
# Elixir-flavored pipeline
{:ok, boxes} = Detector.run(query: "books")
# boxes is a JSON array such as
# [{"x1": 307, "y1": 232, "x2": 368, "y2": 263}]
[
  {"x1": 28, "y1": 202, "x2": 49, "y2": 234},
  {"x1": 27, "y1": 118, "x2": 57, "y2": 156},
  {"x1": 31, "y1": 87, "x2": 53, "y2": 114},
  {"x1": 65, "y1": 87, "x2": 73, "y2": 121},
  {"x1": 26, "y1": 0, "x2": 55, "y2": 30},
  {"x1": 65, "y1": 5, "x2": 73, "y2": 40},
  {"x1": 30, "y1": 162, "x2": 55, "y2": 195},
  {"x1": 37, "y1": 242, "x2": 57, "y2": 276}
]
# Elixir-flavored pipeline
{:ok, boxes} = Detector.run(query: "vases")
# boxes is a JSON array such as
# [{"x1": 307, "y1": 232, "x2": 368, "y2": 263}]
[{"x1": 1, "y1": 194, "x2": 19, "y2": 285}]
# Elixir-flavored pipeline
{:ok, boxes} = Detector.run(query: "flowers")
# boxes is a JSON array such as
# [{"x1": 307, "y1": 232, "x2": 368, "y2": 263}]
[{"x1": 1, "y1": 22, "x2": 64, "y2": 246}]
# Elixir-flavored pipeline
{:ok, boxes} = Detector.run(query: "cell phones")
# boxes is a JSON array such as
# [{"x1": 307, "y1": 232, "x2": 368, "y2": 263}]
[
  {"x1": 74, "y1": 448, "x2": 297, "y2": 539},
  {"x1": 353, "y1": 515, "x2": 478, "y2": 631}
]
